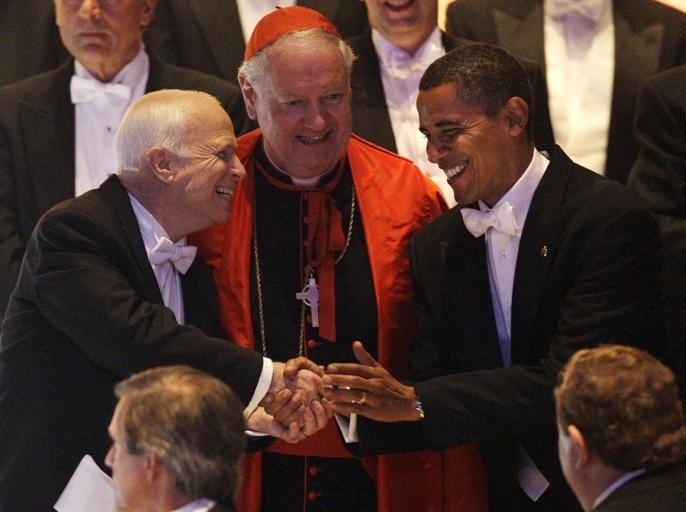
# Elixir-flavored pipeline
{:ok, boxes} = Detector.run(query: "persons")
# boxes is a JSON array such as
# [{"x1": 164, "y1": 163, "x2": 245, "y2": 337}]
[
  {"x1": 105, "y1": 365, "x2": 244, "y2": 512},
  {"x1": 0, "y1": 89, "x2": 335, "y2": 512},
  {"x1": 182, "y1": 5, "x2": 488, "y2": 512},
  {"x1": 344, "y1": 2, "x2": 685, "y2": 346},
  {"x1": 261, "y1": 41, "x2": 674, "y2": 511},
  {"x1": 555, "y1": 347, "x2": 686, "y2": 512},
  {"x1": 0, "y1": 1, "x2": 369, "y2": 308}
]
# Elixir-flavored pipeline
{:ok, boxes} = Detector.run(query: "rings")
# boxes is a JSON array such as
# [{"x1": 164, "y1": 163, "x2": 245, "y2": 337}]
[
  {"x1": 303, "y1": 431, "x2": 312, "y2": 436},
  {"x1": 357, "y1": 392, "x2": 367, "y2": 404}
]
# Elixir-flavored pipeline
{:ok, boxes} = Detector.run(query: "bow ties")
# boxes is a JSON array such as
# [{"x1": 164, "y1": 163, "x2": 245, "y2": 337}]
[
  {"x1": 542, "y1": 0, "x2": 607, "y2": 22},
  {"x1": 147, "y1": 236, "x2": 199, "y2": 277},
  {"x1": 459, "y1": 202, "x2": 516, "y2": 240},
  {"x1": 385, "y1": 40, "x2": 443, "y2": 79},
  {"x1": 65, "y1": 75, "x2": 133, "y2": 116}
]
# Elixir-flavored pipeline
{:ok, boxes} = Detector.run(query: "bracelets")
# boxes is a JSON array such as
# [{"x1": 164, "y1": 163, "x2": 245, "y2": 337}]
[{"x1": 415, "y1": 398, "x2": 424, "y2": 419}]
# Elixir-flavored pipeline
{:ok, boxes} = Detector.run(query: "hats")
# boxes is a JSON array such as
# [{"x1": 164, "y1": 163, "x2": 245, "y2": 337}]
[{"x1": 243, "y1": 3, "x2": 346, "y2": 64}]
